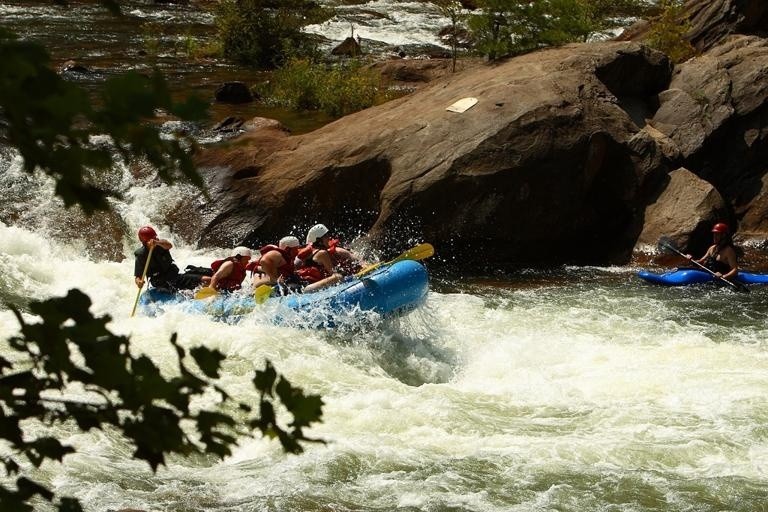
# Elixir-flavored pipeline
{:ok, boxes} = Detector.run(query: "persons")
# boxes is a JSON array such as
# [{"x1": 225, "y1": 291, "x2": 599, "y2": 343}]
[
  {"x1": 686, "y1": 223, "x2": 738, "y2": 280},
  {"x1": 134, "y1": 221, "x2": 346, "y2": 300}
]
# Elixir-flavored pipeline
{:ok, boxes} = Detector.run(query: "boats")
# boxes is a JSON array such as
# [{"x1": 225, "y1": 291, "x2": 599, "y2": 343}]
[
  {"x1": 638, "y1": 270, "x2": 767, "y2": 287},
  {"x1": 140, "y1": 259, "x2": 427, "y2": 330}
]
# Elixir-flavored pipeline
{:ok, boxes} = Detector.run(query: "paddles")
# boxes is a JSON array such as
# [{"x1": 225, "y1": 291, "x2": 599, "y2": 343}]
[
  {"x1": 255, "y1": 279, "x2": 288, "y2": 305},
  {"x1": 657, "y1": 236, "x2": 750, "y2": 292},
  {"x1": 345, "y1": 243, "x2": 435, "y2": 280},
  {"x1": 196, "y1": 287, "x2": 220, "y2": 300}
]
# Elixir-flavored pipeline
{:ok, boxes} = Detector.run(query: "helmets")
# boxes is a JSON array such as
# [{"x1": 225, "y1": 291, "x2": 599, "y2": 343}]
[
  {"x1": 231, "y1": 246, "x2": 253, "y2": 260},
  {"x1": 138, "y1": 226, "x2": 157, "y2": 241},
  {"x1": 304, "y1": 223, "x2": 329, "y2": 245},
  {"x1": 276, "y1": 236, "x2": 302, "y2": 251},
  {"x1": 711, "y1": 222, "x2": 729, "y2": 235}
]
